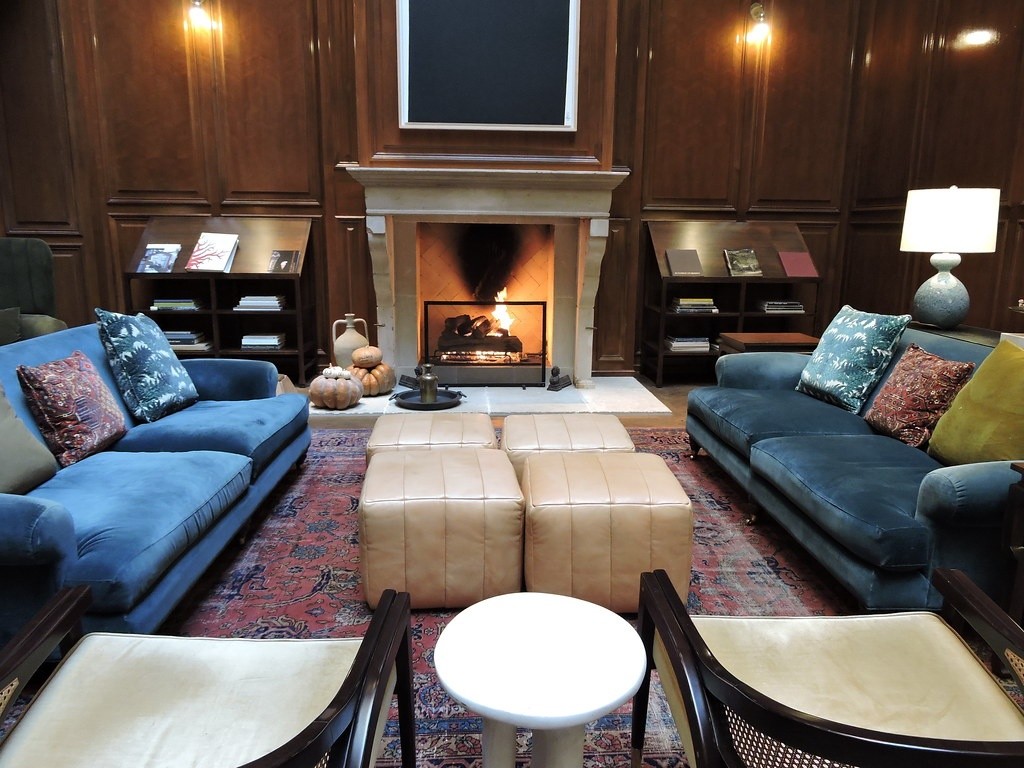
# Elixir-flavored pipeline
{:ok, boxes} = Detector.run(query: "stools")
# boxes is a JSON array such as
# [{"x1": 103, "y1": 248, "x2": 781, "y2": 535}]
[
  {"x1": 366, "y1": 413, "x2": 499, "y2": 468},
  {"x1": 522, "y1": 451, "x2": 694, "y2": 616},
  {"x1": 358, "y1": 449, "x2": 526, "y2": 611},
  {"x1": 500, "y1": 412, "x2": 636, "y2": 491}
]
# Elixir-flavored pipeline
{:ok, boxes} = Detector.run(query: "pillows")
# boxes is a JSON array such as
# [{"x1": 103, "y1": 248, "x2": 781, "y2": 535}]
[
  {"x1": 16, "y1": 349, "x2": 129, "y2": 469},
  {"x1": 927, "y1": 340, "x2": 1024, "y2": 464},
  {"x1": 862, "y1": 342, "x2": 976, "y2": 447},
  {"x1": 0, "y1": 390, "x2": 56, "y2": 497},
  {"x1": 94, "y1": 304, "x2": 200, "y2": 425},
  {"x1": 0, "y1": 307, "x2": 22, "y2": 346},
  {"x1": 791, "y1": 304, "x2": 913, "y2": 416}
]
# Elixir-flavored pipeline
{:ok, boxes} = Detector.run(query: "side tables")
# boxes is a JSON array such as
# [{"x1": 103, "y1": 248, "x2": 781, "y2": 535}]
[{"x1": 718, "y1": 331, "x2": 821, "y2": 356}]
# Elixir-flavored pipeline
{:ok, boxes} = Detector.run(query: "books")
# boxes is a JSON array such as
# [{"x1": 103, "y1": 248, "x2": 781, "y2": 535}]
[
  {"x1": 664, "y1": 335, "x2": 710, "y2": 353},
  {"x1": 232, "y1": 295, "x2": 284, "y2": 312},
  {"x1": 153, "y1": 296, "x2": 206, "y2": 311},
  {"x1": 669, "y1": 298, "x2": 720, "y2": 314},
  {"x1": 746, "y1": 293, "x2": 806, "y2": 314},
  {"x1": 268, "y1": 250, "x2": 301, "y2": 273},
  {"x1": 238, "y1": 332, "x2": 289, "y2": 350},
  {"x1": 184, "y1": 231, "x2": 240, "y2": 274},
  {"x1": 664, "y1": 249, "x2": 704, "y2": 277},
  {"x1": 778, "y1": 252, "x2": 818, "y2": 277},
  {"x1": 162, "y1": 328, "x2": 211, "y2": 351},
  {"x1": 722, "y1": 247, "x2": 763, "y2": 277},
  {"x1": 136, "y1": 243, "x2": 181, "y2": 273}
]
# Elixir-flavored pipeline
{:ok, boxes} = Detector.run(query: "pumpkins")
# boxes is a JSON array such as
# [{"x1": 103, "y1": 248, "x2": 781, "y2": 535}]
[{"x1": 309, "y1": 345, "x2": 397, "y2": 411}]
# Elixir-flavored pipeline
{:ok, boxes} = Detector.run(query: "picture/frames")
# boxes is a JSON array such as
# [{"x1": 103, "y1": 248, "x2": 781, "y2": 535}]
[{"x1": 396, "y1": 0, "x2": 582, "y2": 136}]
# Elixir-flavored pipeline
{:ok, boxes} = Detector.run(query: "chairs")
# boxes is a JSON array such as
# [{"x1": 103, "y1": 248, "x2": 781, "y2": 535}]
[
  {"x1": 629, "y1": 568, "x2": 1024, "y2": 768},
  {"x1": 0, "y1": 236, "x2": 69, "y2": 346},
  {"x1": 0, "y1": 584, "x2": 416, "y2": 768}
]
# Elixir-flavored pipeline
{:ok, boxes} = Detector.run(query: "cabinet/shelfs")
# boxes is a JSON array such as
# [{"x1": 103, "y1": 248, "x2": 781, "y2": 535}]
[
  {"x1": 638, "y1": 217, "x2": 820, "y2": 387},
  {"x1": 123, "y1": 215, "x2": 319, "y2": 388}
]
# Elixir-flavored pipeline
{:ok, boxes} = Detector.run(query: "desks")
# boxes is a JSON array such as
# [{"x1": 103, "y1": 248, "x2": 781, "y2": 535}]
[{"x1": 431, "y1": 593, "x2": 646, "y2": 768}]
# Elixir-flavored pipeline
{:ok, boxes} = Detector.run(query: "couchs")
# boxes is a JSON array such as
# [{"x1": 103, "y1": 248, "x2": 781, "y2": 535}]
[
  {"x1": 0, "y1": 319, "x2": 311, "y2": 634},
  {"x1": 680, "y1": 326, "x2": 1024, "y2": 615}
]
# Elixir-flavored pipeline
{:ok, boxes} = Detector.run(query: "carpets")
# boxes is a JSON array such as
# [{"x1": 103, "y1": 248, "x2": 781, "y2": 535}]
[{"x1": 0, "y1": 425, "x2": 1024, "y2": 768}]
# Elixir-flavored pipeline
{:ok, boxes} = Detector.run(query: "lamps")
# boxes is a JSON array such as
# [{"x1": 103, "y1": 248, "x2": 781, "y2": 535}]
[
  {"x1": 897, "y1": 183, "x2": 1002, "y2": 332},
  {"x1": 192, "y1": 0, "x2": 204, "y2": 7},
  {"x1": 750, "y1": 3, "x2": 767, "y2": 25}
]
